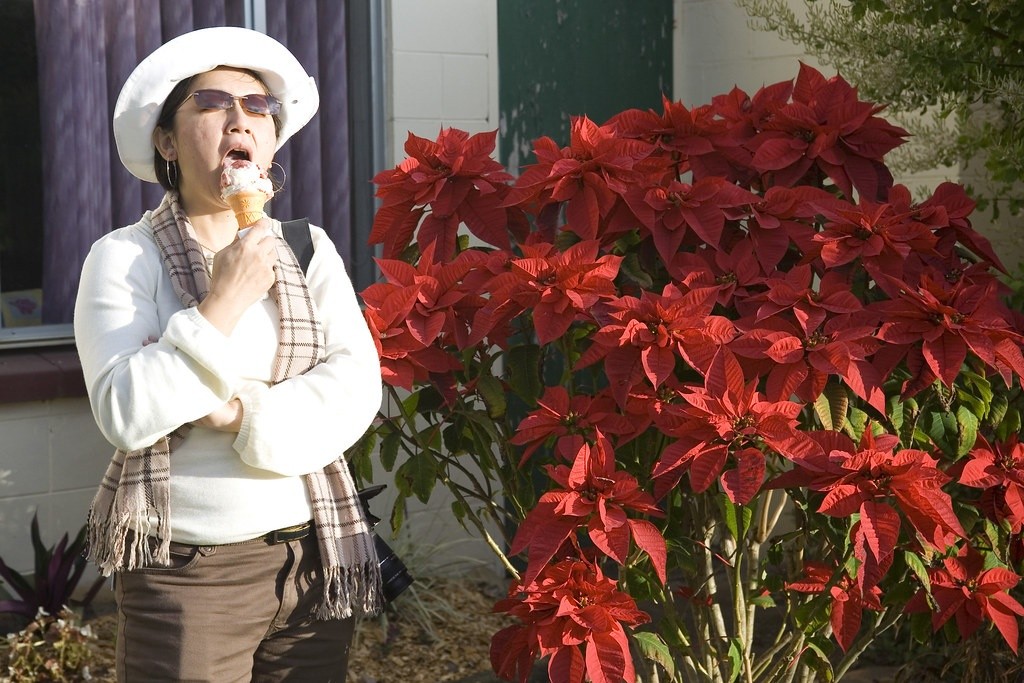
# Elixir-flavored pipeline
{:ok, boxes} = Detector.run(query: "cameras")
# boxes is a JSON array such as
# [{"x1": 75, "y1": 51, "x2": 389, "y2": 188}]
[{"x1": 355, "y1": 484, "x2": 414, "y2": 600}]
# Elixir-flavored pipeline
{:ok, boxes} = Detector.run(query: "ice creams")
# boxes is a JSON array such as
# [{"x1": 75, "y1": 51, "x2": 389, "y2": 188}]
[{"x1": 220, "y1": 158, "x2": 273, "y2": 231}]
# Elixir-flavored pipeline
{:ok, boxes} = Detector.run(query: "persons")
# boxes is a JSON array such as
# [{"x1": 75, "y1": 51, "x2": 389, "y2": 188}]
[{"x1": 72, "y1": 26, "x2": 383, "y2": 683}]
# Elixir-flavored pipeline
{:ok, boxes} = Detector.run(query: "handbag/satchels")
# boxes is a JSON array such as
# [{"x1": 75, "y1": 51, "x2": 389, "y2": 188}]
[{"x1": 339, "y1": 484, "x2": 414, "y2": 603}]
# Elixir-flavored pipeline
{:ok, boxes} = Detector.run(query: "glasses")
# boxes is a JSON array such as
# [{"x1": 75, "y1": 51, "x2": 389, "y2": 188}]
[{"x1": 175, "y1": 89, "x2": 282, "y2": 115}]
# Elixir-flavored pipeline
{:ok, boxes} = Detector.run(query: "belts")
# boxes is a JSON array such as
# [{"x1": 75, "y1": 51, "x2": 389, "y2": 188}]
[{"x1": 272, "y1": 524, "x2": 317, "y2": 545}]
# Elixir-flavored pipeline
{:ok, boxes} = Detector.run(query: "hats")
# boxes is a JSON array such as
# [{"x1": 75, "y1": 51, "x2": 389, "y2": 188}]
[{"x1": 112, "y1": 26, "x2": 321, "y2": 184}]
